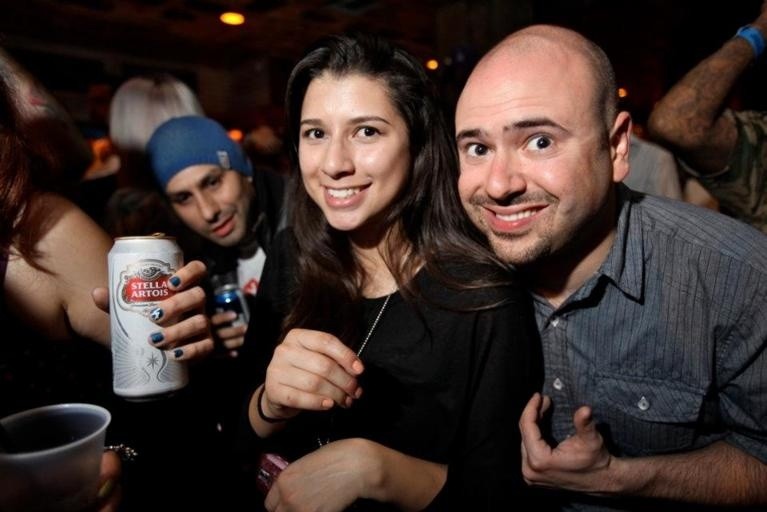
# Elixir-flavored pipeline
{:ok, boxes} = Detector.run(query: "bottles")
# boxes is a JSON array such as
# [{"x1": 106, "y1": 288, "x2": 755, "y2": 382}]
[
  {"x1": 108, "y1": 232, "x2": 188, "y2": 403},
  {"x1": 215, "y1": 283, "x2": 251, "y2": 327}
]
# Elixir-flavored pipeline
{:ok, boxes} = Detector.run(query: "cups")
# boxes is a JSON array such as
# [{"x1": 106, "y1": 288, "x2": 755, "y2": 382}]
[{"x1": 0, "y1": 401, "x2": 112, "y2": 511}]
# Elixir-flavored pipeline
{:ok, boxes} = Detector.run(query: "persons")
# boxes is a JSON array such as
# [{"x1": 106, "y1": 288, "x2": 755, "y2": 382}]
[
  {"x1": 227, "y1": 30, "x2": 544, "y2": 511},
  {"x1": 145, "y1": 114, "x2": 278, "y2": 357},
  {"x1": 0, "y1": 62, "x2": 215, "y2": 363},
  {"x1": 93, "y1": 75, "x2": 204, "y2": 249},
  {"x1": 454, "y1": 23, "x2": 767, "y2": 511},
  {"x1": 649, "y1": 0, "x2": 766, "y2": 225}
]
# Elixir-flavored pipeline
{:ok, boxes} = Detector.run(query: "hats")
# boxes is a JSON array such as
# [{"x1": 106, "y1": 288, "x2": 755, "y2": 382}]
[{"x1": 145, "y1": 114, "x2": 254, "y2": 189}]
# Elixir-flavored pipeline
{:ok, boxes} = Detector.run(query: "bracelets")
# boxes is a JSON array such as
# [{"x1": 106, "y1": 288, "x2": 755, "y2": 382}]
[
  {"x1": 733, "y1": 25, "x2": 763, "y2": 55},
  {"x1": 257, "y1": 384, "x2": 303, "y2": 425}
]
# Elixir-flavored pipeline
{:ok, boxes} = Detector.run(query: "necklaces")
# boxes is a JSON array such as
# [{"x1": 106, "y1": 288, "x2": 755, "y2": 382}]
[{"x1": 356, "y1": 245, "x2": 412, "y2": 358}]
